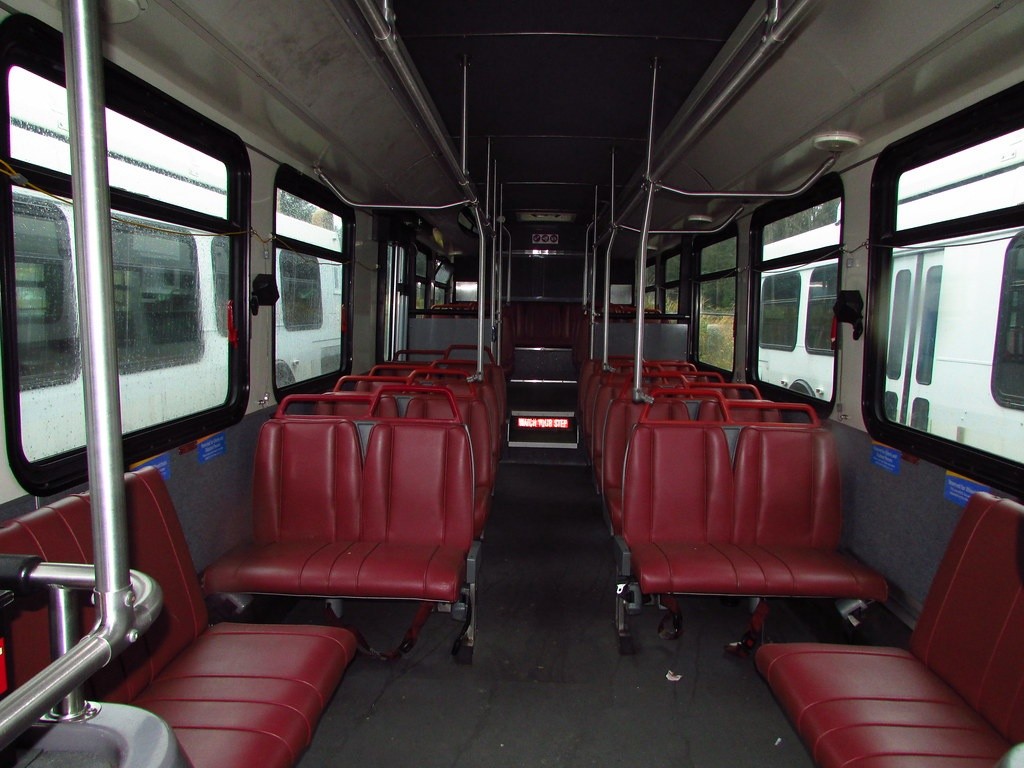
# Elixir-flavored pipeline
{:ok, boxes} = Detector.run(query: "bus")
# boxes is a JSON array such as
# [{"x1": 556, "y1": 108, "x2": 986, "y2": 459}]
[
  {"x1": 758, "y1": 129, "x2": 1024, "y2": 467},
  {"x1": 7, "y1": 66, "x2": 342, "y2": 462}
]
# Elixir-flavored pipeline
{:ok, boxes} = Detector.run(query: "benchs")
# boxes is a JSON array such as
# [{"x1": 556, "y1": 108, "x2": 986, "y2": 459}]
[{"x1": 0, "y1": 296, "x2": 1024, "y2": 768}]
